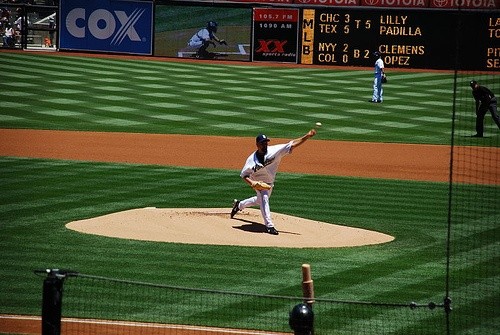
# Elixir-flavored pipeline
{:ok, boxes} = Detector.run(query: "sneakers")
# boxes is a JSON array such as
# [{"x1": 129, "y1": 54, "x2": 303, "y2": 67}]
[
  {"x1": 231, "y1": 199, "x2": 241, "y2": 218},
  {"x1": 264, "y1": 226, "x2": 278, "y2": 236}
]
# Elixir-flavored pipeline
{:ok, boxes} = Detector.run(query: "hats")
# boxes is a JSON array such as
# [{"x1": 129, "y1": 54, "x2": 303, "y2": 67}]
[
  {"x1": 470, "y1": 81, "x2": 476, "y2": 86},
  {"x1": 256, "y1": 135, "x2": 270, "y2": 143}
]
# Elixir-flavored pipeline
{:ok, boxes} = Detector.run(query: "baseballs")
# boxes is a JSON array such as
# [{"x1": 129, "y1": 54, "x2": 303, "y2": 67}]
[{"x1": 315, "y1": 121, "x2": 322, "y2": 127}]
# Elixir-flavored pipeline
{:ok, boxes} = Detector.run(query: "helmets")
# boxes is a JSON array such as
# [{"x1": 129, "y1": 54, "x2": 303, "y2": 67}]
[
  {"x1": 208, "y1": 21, "x2": 217, "y2": 27},
  {"x1": 373, "y1": 51, "x2": 380, "y2": 56}
]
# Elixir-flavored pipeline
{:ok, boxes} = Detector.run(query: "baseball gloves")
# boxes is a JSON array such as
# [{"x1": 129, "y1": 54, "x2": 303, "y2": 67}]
[{"x1": 381, "y1": 75, "x2": 386, "y2": 83}]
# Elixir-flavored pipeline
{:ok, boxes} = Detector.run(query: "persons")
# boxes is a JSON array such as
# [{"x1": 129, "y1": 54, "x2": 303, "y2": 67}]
[
  {"x1": 231, "y1": 129, "x2": 316, "y2": 234},
  {"x1": 2, "y1": 23, "x2": 14, "y2": 47},
  {"x1": 187, "y1": 21, "x2": 228, "y2": 58},
  {"x1": 470, "y1": 80, "x2": 500, "y2": 137},
  {"x1": 14, "y1": 8, "x2": 25, "y2": 30},
  {"x1": 369, "y1": 51, "x2": 387, "y2": 103},
  {"x1": 49, "y1": 17, "x2": 55, "y2": 47}
]
